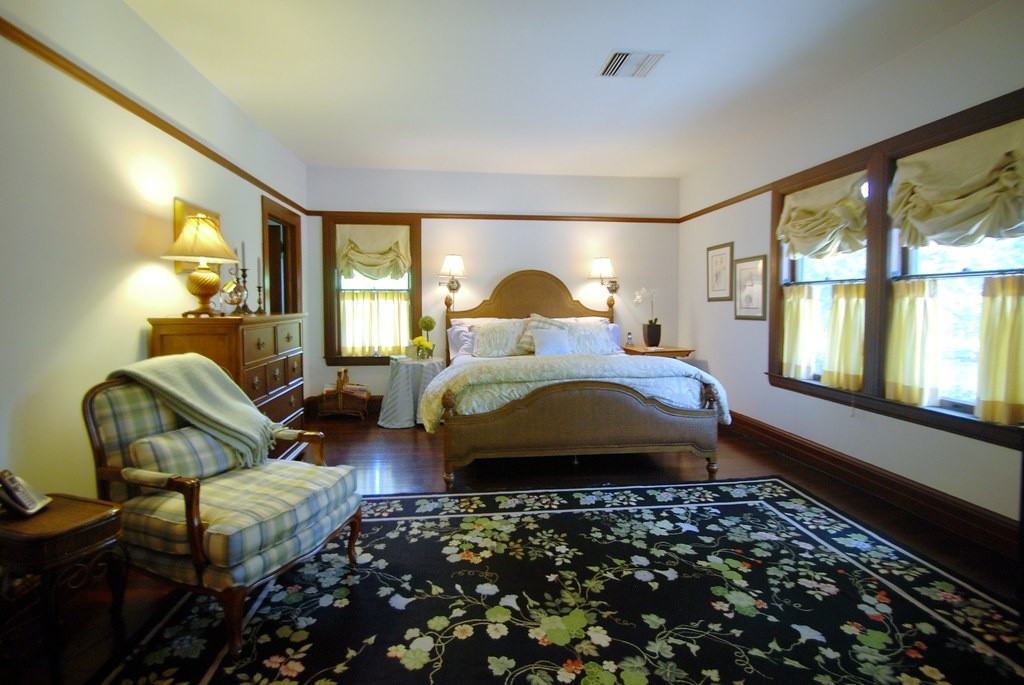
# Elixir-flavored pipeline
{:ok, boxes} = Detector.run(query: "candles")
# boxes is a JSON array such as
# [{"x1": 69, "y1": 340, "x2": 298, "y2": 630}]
[{"x1": 234, "y1": 239, "x2": 262, "y2": 286}]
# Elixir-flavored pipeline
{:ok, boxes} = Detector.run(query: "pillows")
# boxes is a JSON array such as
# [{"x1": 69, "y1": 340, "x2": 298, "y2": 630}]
[
  {"x1": 129, "y1": 425, "x2": 240, "y2": 494},
  {"x1": 468, "y1": 317, "x2": 613, "y2": 357}
]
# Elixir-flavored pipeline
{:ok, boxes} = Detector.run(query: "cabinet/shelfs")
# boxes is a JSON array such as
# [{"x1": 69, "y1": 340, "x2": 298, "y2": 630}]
[{"x1": 146, "y1": 313, "x2": 309, "y2": 461}]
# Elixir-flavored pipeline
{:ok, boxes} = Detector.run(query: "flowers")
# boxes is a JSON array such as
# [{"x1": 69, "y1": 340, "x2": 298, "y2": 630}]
[
  {"x1": 412, "y1": 335, "x2": 434, "y2": 350},
  {"x1": 633, "y1": 288, "x2": 658, "y2": 325}
]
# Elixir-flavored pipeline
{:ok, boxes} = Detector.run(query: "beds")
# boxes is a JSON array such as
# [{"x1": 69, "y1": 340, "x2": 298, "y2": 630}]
[{"x1": 442, "y1": 270, "x2": 719, "y2": 491}]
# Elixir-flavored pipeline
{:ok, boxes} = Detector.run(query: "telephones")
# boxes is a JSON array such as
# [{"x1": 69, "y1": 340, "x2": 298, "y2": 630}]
[{"x1": 0, "y1": 469, "x2": 54, "y2": 516}]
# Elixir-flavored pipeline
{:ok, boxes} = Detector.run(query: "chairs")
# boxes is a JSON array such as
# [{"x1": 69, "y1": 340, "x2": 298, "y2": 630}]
[{"x1": 82, "y1": 364, "x2": 362, "y2": 658}]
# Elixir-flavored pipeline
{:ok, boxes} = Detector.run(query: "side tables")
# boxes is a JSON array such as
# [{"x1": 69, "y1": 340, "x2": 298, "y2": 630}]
[
  {"x1": 620, "y1": 346, "x2": 696, "y2": 359},
  {"x1": 317, "y1": 383, "x2": 368, "y2": 420}
]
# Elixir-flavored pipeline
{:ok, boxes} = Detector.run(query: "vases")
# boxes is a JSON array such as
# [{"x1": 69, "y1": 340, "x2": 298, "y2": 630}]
[
  {"x1": 643, "y1": 324, "x2": 661, "y2": 347},
  {"x1": 417, "y1": 348, "x2": 428, "y2": 359}
]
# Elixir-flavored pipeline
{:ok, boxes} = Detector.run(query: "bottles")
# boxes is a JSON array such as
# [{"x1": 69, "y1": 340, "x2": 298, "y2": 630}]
[{"x1": 628, "y1": 332, "x2": 632, "y2": 346}]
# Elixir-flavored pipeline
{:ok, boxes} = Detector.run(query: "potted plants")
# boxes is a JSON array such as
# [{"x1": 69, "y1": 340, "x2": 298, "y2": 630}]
[{"x1": 417, "y1": 316, "x2": 436, "y2": 359}]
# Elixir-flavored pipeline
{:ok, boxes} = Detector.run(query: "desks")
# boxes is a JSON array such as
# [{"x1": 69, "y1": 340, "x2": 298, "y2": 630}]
[
  {"x1": 390, "y1": 358, "x2": 445, "y2": 420},
  {"x1": 0, "y1": 494, "x2": 125, "y2": 672}
]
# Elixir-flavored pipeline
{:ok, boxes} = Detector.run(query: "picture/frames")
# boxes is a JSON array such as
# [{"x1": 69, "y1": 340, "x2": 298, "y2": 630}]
[
  {"x1": 174, "y1": 197, "x2": 223, "y2": 276},
  {"x1": 704, "y1": 241, "x2": 767, "y2": 320}
]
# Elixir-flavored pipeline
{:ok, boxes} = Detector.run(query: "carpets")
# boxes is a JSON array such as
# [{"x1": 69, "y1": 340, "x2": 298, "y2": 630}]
[{"x1": 74, "y1": 473, "x2": 1024, "y2": 685}]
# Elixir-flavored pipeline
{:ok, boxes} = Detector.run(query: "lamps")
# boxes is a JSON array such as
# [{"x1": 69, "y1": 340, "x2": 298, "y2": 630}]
[
  {"x1": 438, "y1": 254, "x2": 468, "y2": 293},
  {"x1": 586, "y1": 256, "x2": 619, "y2": 293},
  {"x1": 159, "y1": 216, "x2": 242, "y2": 317}
]
